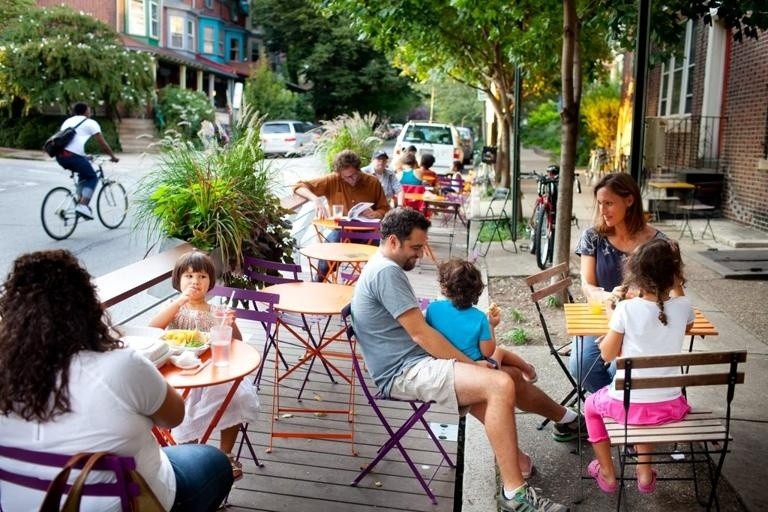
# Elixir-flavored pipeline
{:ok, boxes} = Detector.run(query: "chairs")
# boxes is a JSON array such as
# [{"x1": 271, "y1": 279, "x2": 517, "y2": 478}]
[
  {"x1": 580, "y1": 350, "x2": 747, "y2": 512},
  {"x1": 678, "y1": 182, "x2": 723, "y2": 254},
  {"x1": 471, "y1": 187, "x2": 518, "y2": 257},
  {"x1": 523, "y1": 263, "x2": 586, "y2": 430},
  {"x1": 646, "y1": 167, "x2": 678, "y2": 226}
]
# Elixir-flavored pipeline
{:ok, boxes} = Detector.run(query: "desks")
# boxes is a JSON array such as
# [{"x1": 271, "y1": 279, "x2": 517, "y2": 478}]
[
  {"x1": 563, "y1": 303, "x2": 718, "y2": 507},
  {"x1": 648, "y1": 182, "x2": 696, "y2": 243}
]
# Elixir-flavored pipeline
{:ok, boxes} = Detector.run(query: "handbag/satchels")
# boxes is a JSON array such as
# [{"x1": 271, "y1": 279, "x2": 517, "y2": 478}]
[{"x1": 43, "y1": 127, "x2": 75, "y2": 158}]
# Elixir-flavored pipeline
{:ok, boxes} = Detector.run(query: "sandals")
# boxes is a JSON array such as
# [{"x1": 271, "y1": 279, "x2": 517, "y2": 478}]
[{"x1": 226, "y1": 452, "x2": 243, "y2": 479}]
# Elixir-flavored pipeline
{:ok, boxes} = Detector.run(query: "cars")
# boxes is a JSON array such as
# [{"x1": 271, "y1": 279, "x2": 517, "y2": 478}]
[
  {"x1": 377, "y1": 122, "x2": 402, "y2": 140},
  {"x1": 458, "y1": 126, "x2": 473, "y2": 160}
]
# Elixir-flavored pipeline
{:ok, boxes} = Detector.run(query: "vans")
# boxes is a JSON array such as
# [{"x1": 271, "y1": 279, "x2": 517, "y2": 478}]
[{"x1": 258, "y1": 120, "x2": 310, "y2": 157}]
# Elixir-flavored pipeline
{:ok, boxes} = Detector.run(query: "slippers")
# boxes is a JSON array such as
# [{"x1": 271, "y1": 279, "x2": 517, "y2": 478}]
[
  {"x1": 636, "y1": 465, "x2": 657, "y2": 493},
  {"x1": 587, "y1": 459, "x2": 617, "y2": 493},
  {"x1": 522, "y1": 452, "x2": 534, "y2": 478},
  {"x1": 521, "y1": 360, "x2": 537, "y2": 383}
]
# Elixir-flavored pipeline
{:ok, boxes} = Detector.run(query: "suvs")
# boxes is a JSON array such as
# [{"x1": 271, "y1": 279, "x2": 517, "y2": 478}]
[{"x1": 391, "y1": 119, "x2": 463, "y2": 176}]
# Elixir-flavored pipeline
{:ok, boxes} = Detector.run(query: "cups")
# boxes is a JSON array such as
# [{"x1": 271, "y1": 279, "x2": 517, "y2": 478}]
[
  {"x1": 590, "y1": 289, "x2": 604, "y2": 314},
  {"x1": 212, "y1": 305, "x2": 235, "y2": 325},
  {"x1": 333, "y1": 204, "x2": 344, "y2": 223},
  {"x1": 210, "y1": 324, "x2": 233, "y2": 364}
]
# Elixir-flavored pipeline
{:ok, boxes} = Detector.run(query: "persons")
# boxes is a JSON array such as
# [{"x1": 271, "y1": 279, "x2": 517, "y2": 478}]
[
  {"x1": 360, "y1": 150, "x2": 405, "y2": 211},
  {"x1": 0, "y1": 249, "x2": 232, "y2": 512},
  {"x1": 440, "y1": 160, "x2": 465, "y2": 197},
  {"x1": 426, "y1": 259, "x2": 539, "y2": 384},
  {"x1": 149, "y1": 251, "x2": 243, "y2": 479},
  {"x1": 584, "y1": 238, "x2": 695, "y2": 494},
  {"x1": 394, "y1": 146, "x2": 440, "y2": 224},
  {"x1": 569, "y1": 172, "x2": 671, "y2": 395},
  {"x1": 349, "y1": 208, "x2": 588, "y2": 512},
  {"x1": 55, "y1": 103, "x2": 120, "y2": 220},
  {"x1": 529, "y1": 165, "x2": 560, "y2": 266},
  {"x1": 292, "y1": 150, "x2": 392, "y2": 283}
]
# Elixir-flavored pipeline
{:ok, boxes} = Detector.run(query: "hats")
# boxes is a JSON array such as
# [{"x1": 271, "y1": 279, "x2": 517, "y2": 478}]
[{"x1": 373, "y1": 149, "x2": 388, "y2": 160}]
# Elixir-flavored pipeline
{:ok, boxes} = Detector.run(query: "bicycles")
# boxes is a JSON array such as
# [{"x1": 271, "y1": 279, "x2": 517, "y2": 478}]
[
  {"x1": 520, "y1": 169, "x2": 581, "y2": 272},
  {"x1": 40, "y1": 156, "x2": 128, "y2": 241}
]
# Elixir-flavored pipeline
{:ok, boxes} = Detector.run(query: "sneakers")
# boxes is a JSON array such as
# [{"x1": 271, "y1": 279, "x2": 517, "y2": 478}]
[
  {"x1": 552, "y1": 408, "x2": 589, "y2": 442},
  {"x1": 74, "y1": 202, "x2": 94, "y2": 220},
  {"x1": 499, "y1": 479, "x2": 570, "y2": 512}
]
